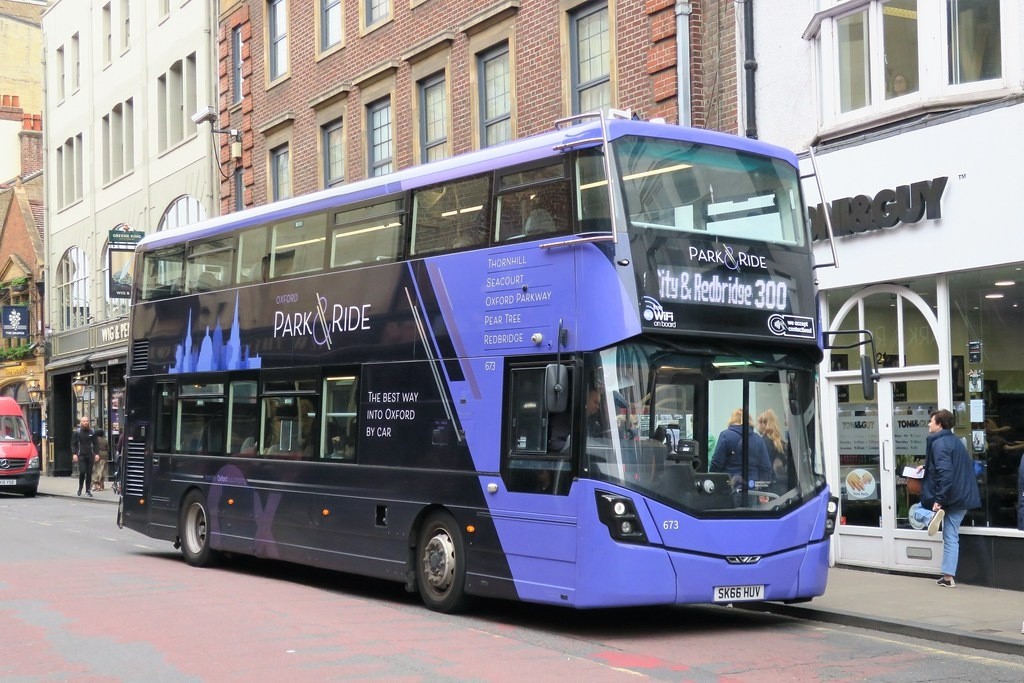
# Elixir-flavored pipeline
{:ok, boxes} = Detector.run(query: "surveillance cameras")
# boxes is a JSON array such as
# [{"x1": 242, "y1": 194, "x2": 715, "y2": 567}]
[{"x1": 191, "y1": 106, "x2": 215, "y2": 124}]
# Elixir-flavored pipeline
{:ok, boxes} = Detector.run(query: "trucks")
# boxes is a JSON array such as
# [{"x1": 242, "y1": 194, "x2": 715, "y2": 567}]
[{"x1": 0, "y1": 397, "x2": 41, "y2": 498}]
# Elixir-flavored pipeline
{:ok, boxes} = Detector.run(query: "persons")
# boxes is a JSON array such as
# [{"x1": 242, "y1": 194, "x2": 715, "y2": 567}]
[
  {"x1": 550, "y1": 386, "x2": 607, "y2": 453},
  {"x1": 973, "y1": 420, "x2": 1024, "y2": 451},
  {"x1": 709, "y1": 407, "x2": 792, "y2": 511},
  {"x1": 248, "y1": 399, "x2": 315, "y2": 446},
  {"x1": 914, "y1": 410, "x2": 981, "y2": 588},
  {"x1": 91, "y1": 428, "x2": 110, "y2": 491},
  {"x1": 117, "y1": 433, "x2": 124, "y2": 493},
  {"x1": 71, "y1": 416, "x2": 100, "y2": 497}
]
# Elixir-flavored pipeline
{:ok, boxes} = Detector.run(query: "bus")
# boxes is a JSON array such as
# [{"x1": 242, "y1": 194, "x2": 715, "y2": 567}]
[{"x1": 118, "y1": 109, "x2": 882, "y2": 613}]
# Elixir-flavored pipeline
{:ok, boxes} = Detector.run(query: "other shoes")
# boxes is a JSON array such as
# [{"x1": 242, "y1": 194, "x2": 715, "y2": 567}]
[
  {"x1": 77, "y1": 490, "x2": 81, "y2": 496},
  {"x1": 85, "y1": 492, "x2": 92, "y2": 497}
]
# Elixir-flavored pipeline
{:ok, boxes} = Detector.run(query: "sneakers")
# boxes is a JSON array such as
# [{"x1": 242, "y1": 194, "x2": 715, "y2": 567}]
[
  {"x1": 936, "y1": 577, "x2": 956, "y2": 588},
  {"x1": 927, "y1": 509, "x2": 945, "y2": 536}
]
